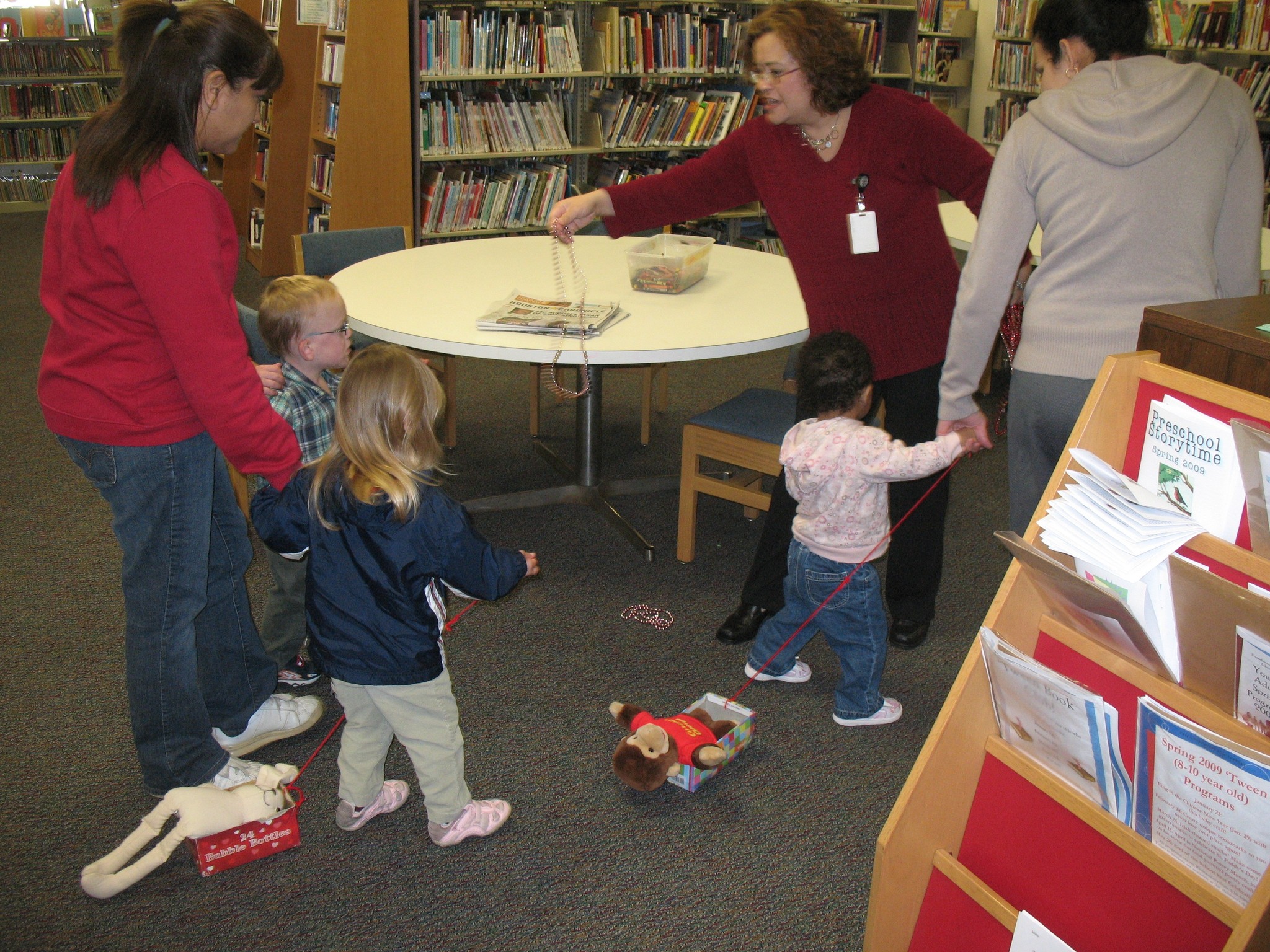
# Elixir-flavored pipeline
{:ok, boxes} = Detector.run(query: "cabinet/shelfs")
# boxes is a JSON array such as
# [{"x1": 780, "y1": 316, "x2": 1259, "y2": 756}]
[{"x1": 0, "y1": 0, "x2": 1270, "y2": 952}]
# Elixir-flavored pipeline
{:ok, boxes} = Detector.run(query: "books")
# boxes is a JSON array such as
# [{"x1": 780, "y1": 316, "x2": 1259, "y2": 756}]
[{"x1": 0, "y1": 0, "x2": 1270, "y2": 260}]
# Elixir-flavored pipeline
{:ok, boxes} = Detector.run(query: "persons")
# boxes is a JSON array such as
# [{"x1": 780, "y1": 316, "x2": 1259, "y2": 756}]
[
  {"x1": 546, "y1": 0, "x2": 1031, "y2": 644},
  {"x1": 936, "y1": 0, "x2": 1263, "y2": 538},
  {"x1": 37, "y1": 0, "x2": 541, "y2": 847},
  {"x1": 745, "y1": 326, "x2": 981, "y2": 726}
]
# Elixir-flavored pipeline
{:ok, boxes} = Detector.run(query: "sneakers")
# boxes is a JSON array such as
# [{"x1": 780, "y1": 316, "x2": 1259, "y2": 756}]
[
  {"x1": 210, "y1": 693, "x2": 323, "y2": 757},
  {"x1": 834, "y1": 697, "x2": 902, "y2": 726},
  {"x1": 745, "y1": 656, "x2": 811, "y2": 683},
  {"x1": 428, "y1": 798, "x2": 511, "y2": 847},
  {"x1": 275, "y1": 653, "x2": 321, "y2": 688},
  {"x1": 145, "y1": 753, "x2": 263, "y2": 798},
  {"x1": 336, "y1": 779, "x2": 409, "y2": 830}
]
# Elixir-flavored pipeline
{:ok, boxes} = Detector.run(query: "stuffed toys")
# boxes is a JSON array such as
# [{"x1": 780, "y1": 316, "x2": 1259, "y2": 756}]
[{"x1": 608, "y1": 702, "x2": 738, "y2": 791}]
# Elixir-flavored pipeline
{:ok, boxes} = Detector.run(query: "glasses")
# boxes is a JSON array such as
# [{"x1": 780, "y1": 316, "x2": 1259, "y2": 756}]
[
  {"x1": 303, "y1": 312, "x2": 350, "y2": 338},
  {"x1": 747, "y1": 65, "x2": 803, "y2": 87}
]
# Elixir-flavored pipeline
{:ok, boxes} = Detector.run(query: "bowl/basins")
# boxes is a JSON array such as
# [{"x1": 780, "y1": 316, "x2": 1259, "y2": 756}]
[{"x1": 624, "y1": 234, "x2": 716, "y2": 295}]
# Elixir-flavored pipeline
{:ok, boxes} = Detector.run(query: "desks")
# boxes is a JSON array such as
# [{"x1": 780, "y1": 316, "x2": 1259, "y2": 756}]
[
  {"x1": 936, "y1": 200, "x2": 1270, "y2": 396},
  {"x1": 325, "y1": 234, "x2": 812, "y2": 565}
]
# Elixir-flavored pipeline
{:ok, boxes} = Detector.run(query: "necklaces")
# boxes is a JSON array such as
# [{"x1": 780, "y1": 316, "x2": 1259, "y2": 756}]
[
  {"x1": 550, "y1": 217, "x2": 590, "y2": 398},
  {"x1": 798, "y1": 107, "x2": 841, "y2": 156}
]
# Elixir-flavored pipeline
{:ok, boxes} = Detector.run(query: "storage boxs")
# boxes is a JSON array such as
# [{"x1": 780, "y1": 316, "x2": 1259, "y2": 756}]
[{"x1": 624, "y1": 233, "x2": 717, "y2": 295}]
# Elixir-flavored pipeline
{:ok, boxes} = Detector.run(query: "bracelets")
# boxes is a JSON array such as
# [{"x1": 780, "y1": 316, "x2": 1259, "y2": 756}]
[{"x1": 1014, "y1": 280, "x2": 1026, "y2": 290}]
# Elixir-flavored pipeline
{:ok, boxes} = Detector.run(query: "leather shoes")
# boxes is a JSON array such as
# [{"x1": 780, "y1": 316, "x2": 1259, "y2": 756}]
[
  {"x1": 715, "y1": 603, "x2": 772, "y2": 644},
  {"x1": 888, "y1": 617, "x2": 930, "y2": 649}
]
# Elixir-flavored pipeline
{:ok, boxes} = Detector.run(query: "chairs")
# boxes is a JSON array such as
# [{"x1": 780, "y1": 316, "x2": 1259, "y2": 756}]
[
  {"x1": 675, "y1": 372, "x2": 890, "y2": 565},
  {"x1": 528, "y1": 218, "x2": 673, "y2": 449},
  {"x1": 289, "y1": 225, "x2": 457, "y2": 449},
  {"x1": 232, "y1": 298, "x2": 287, "y2": 521}
]
